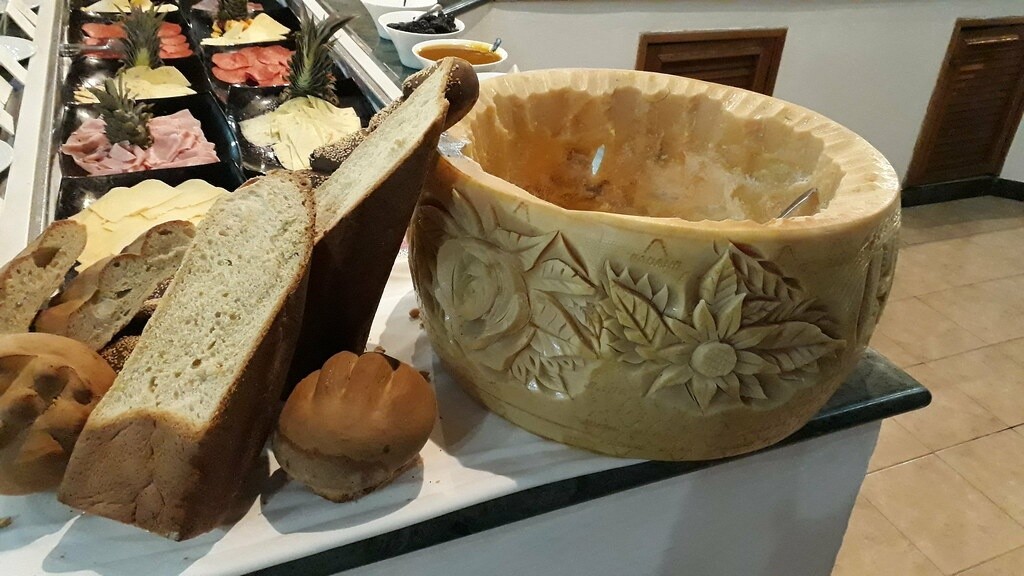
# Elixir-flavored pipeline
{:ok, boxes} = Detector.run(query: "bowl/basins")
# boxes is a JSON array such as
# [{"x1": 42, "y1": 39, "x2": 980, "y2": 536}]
[
  {"x1": 379, "y1": 11, "x2": 466, "y2": 71},
  {"x1": 408, "y1": 66, "x2": 904, "y2": 464},
  {"x1": 412, "y1": 40, "x2": 509, "y2": 71},
  {"x1": 360, "y1": 1, "x2": 438, "y2": 40}
]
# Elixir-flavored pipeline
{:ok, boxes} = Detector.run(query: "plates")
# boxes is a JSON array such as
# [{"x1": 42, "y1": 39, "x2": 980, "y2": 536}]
[
  {"x1": 54, "y1": 0, "x2": 379, "y2": 222},
  {"x1": 0, "y1": 140, "x2": 14, "y2": 173},
  {"x1": 0, "y1": 34, "x2": 36, "y2": 62},
  {"x1": 21, "y1": 0, "x2": 40, "y2": 10}
]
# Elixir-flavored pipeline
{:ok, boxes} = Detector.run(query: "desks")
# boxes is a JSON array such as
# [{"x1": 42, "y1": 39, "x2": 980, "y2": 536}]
[{"x1": 0, "y1": 0, "x2": 931, "y2": 573}]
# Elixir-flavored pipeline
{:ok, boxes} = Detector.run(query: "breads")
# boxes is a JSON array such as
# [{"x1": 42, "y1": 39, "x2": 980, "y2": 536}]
[{"x1": 0, "y1": 56, "x2": 479, "y2": 543}]
